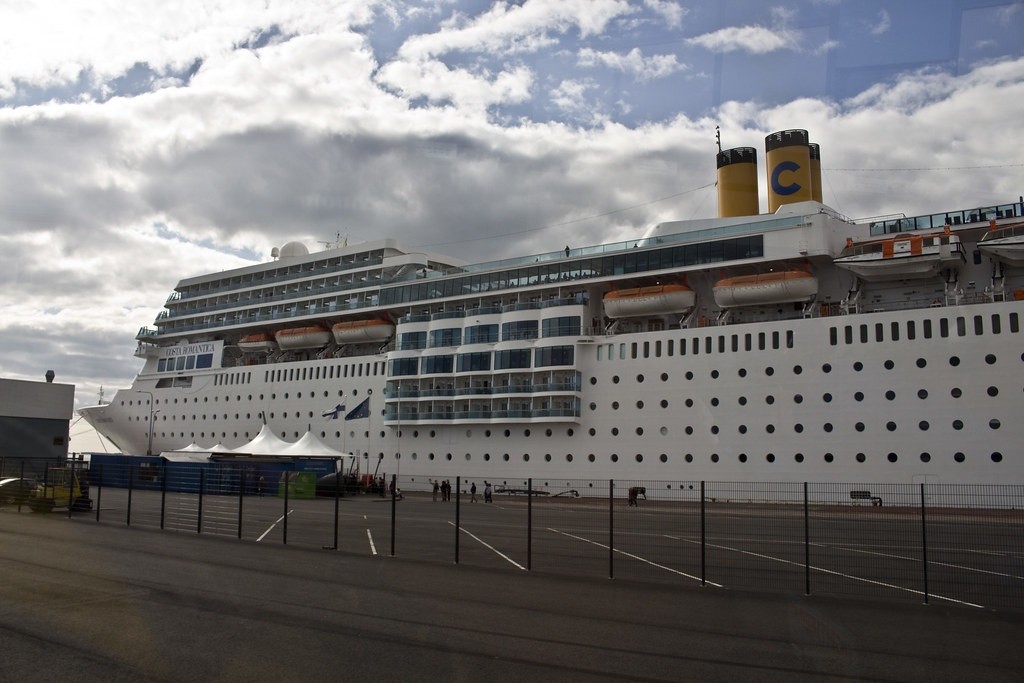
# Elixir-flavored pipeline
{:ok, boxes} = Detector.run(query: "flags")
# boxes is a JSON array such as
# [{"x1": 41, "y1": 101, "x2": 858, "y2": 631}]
[
  {"x1": 345, "y1": 397, "x2": 369, "y2": 420},
  {"x1": 989, "y1": 219, "x2": 997, "y2": 231},
  {"x1": 847, "y1": 238, "x2": 852, "y2": 248},
  {"x1": 944, "y1": 225, "x2": 950, "y2": 234},
  {"x1": 321, "y1": 398, "x2": 345, "y2": 421}
]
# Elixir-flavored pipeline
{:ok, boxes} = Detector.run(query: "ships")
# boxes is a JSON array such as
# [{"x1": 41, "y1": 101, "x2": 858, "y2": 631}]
[{"x1": 75, "y1": 123, "x2": 1022, "y2": 511}]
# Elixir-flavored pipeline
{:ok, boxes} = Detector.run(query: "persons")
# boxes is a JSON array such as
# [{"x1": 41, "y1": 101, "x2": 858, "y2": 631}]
[
  {"x1": 511, "y1": 282, "x2": 514, "y2": 286},
  {"x1": 484, "y1": 481, "x2": 493, "y2": 503},
  {"x1": 545, "y1": 276, "x2": 550, "y2": 280},
  {"x1": 565, "y1": 246, "x2": 570, "y2": 257},
  {"x1": 375, "y1": 476, "x2": 386, "y2": 496},
  {"x1": 634, "y1": 244, "x2": 638, "y2": 247},
  {"x1": 429, "y1": 478, "x2": 451, "y2": 502},
  {"x1": 396, "y1": 488, "x2": 402, "y2": 498},
  {"x1": 423, "y1": 269, "x2": 426, "y2": 278},
  {"x1": 583, "y1": 272, "x2": 586, "y2": 276},
  {"x1": 595, "y1": 271, "x2": 598, "y2": 275},
  {"x1": 471, "y1": 482, "x2": 478, "y2": 503},
  {"x1": 563, "y1": 273, "x2": 568, "y2": 279}
]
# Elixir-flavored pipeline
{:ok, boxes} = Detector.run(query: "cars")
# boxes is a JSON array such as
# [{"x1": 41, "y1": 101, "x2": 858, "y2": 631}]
[
  {"x1": 0, "y1": 477, "x2": 35, "y2": 505},
  {"x1": 30, "y1": 467, "x2": 92, "y2": 511}
]
